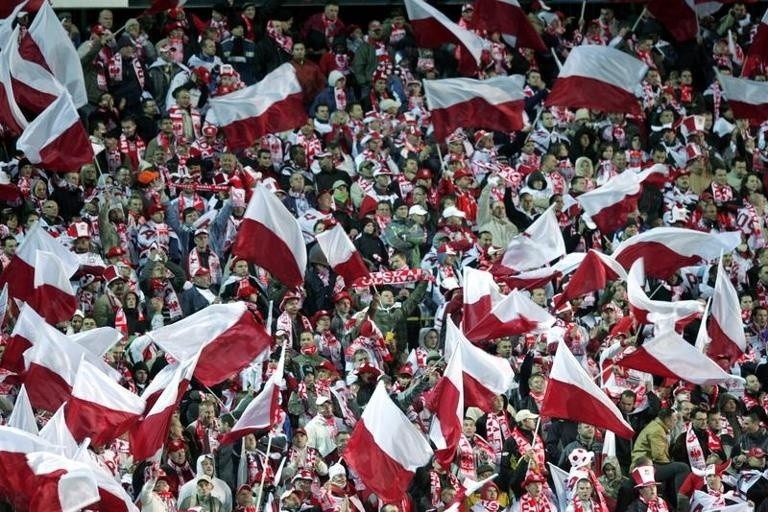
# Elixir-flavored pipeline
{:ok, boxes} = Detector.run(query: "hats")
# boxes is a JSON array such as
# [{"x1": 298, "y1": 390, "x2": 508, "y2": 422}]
[
  {"x1": 18, "y1": 11, "x2": 137, "y2": 365},
  {"x1": 138, "y1": 0, "x2": 768, "y2": 501}
]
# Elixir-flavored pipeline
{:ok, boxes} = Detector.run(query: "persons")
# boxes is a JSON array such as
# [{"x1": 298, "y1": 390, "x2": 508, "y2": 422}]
[{"x1": 0, "y1": 2, "x2": 767, "y2": 512}]
